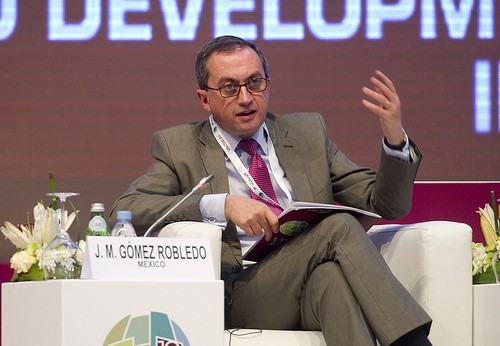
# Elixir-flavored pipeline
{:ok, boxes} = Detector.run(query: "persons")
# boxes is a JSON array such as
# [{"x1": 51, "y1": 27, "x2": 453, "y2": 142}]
[{"x1": 106, "y1": 35, "x2": 433, "y2": 346}]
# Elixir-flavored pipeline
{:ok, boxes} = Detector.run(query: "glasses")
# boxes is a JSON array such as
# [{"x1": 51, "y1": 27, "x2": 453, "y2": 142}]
[{"x1": 205, "y1": 73, "x2": 270, "y2": 98}]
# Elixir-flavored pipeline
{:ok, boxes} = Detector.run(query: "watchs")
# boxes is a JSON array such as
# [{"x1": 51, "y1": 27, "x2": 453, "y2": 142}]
[{"x1": 384, "y1": 134, "x2": 407, "y2": 150}]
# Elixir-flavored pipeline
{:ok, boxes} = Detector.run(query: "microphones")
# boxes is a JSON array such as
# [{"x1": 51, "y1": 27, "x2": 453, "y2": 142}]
[{"x1": 143, "y1": 173, "x2": 215, "y2": 237}]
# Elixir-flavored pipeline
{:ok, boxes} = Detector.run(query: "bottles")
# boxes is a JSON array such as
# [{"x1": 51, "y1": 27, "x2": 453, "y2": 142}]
[
  {"x1": 111, "y1": 211, "x2": 137, "y2": 237},
  {"x1": 85, "y1": 202, "x2": 110, "y2": 240}
]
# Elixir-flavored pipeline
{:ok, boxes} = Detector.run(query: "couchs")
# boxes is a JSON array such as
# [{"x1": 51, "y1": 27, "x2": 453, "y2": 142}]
[{"x1": 159, "y1": 222, "x2": 473, "y2": 346}]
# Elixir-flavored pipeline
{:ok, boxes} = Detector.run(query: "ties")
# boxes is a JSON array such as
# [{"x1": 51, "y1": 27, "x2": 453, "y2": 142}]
[{"x1": 238, "y1": 139, "x2": 284, "y2": 217}]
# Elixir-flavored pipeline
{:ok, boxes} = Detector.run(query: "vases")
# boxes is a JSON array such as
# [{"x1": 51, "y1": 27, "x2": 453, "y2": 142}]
[
  {"x1": 475, "y1": 262, "x2": 500, "y2": 283},
  {"x1": 20, "y1": 263, "x2": 81, "y2": 279}
]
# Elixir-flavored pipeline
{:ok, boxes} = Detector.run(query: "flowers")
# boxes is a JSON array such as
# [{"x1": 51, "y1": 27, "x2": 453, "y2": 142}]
[
  {"x1": 471, "y1": 190, "x2": 500, "y2": 285},
  {"x1": 0, "y1": 170, "x2": 88, "y2": 276}
]
